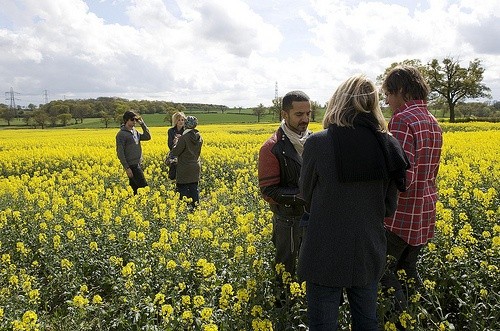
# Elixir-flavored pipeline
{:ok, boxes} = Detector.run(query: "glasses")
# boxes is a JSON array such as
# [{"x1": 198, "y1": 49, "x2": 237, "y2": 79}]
[{"x1": 129, "y1": 118, "x2": 138, "y2": 122}]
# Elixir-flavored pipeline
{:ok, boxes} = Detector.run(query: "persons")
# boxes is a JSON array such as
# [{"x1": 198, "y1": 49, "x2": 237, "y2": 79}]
[
  {"x1": 116, "y1": 111, "x2": 152, "y2": 194},
  {"x1": 381, "y1": 66, "x2": 443, "y2": 320},
  {"x1": 167, "y1": 112, "x2": 185, "y2": 180},
  {"x1": 258, "y1": 90, "x2": 312, "y2": 331},
  {"x1": 165, "y1": 115, "x2": 203, "y2": 214},
  {"x1": 296, "y1": 76, "x2": 411, "y2": 331}
]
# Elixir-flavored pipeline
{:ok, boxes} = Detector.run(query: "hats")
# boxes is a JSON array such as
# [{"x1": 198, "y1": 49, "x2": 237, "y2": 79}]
[{"x1": 184, "y1": 116, "x2": 198, "y2": 128}]
